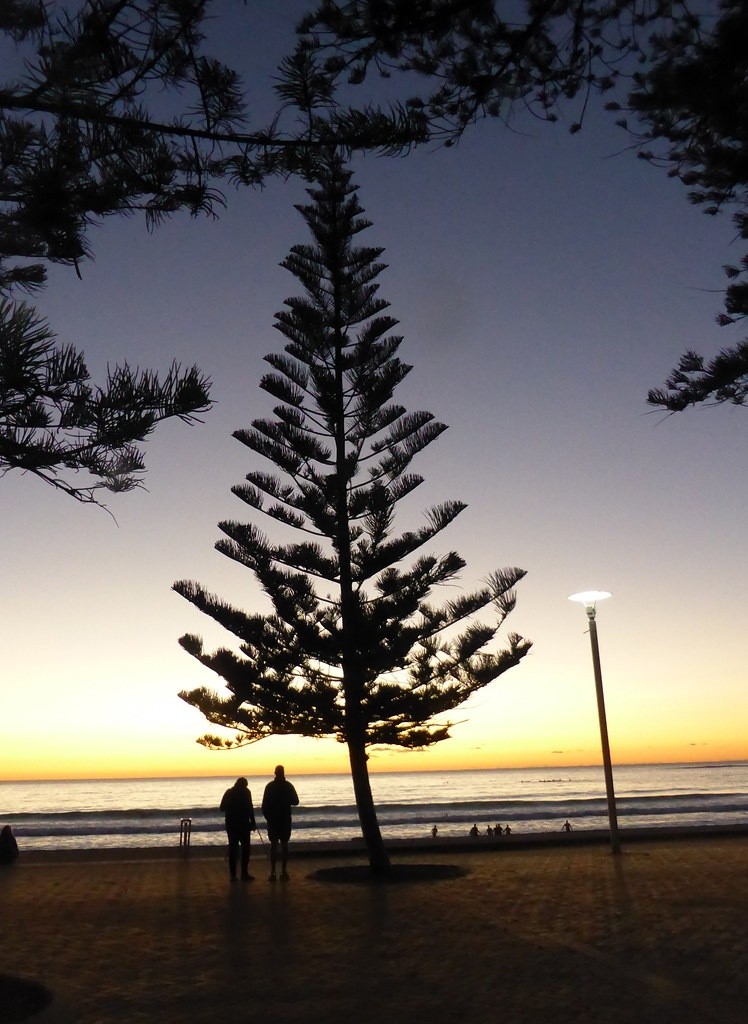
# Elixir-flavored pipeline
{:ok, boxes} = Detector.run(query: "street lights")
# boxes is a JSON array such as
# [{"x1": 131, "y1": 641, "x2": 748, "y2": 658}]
[{"x1": 568, "y1": 588, "x2": 624, "y2": 860}]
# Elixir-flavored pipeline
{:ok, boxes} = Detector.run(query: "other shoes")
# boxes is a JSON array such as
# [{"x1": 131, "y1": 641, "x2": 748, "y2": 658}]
[
  {"x1": 231, "y1": 877, "x2": 238, "y2": 881},
  {"x1": 240, "y1": 875, "x2": 254, "y2": 881},
  {"x1": 270, "y1": 873, "x2": 276, "y2": 880},
  {"x1": 280, "y1": 872, "x2": 289, "y2": 880}
]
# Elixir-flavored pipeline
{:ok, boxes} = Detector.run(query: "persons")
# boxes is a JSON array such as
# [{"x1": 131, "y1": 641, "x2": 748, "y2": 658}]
[
  {"x1": 431, "y1": 825, "x2": 438, "y2": 838},
  {"x1": 262, "y1": 766, "x2": 300, "y2": 880},
  {"x1": 0, "y1": 825, "x2": 19, "y2": 866},
  {"x1": 469, "y1": 824, "x2": 511, "y2": 837},
  {"x1": 220, "y1": 777, "x2": 257, "y2": 883},
  {"x1": 561, "y1": 820, "x2": 573, "y2": 832}
]
